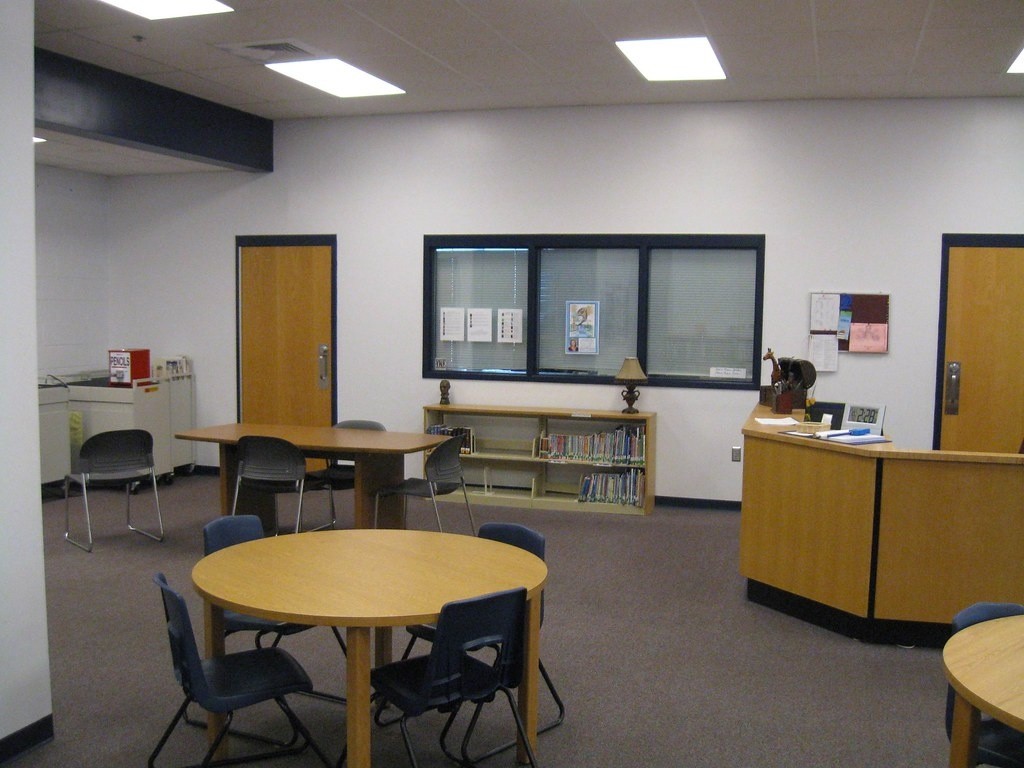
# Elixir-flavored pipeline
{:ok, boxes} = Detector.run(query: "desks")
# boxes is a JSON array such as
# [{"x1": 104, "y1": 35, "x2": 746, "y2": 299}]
[
  {"x1": 173, "y1": 422, "x2": 454, "y2": 530},
  {"x1": 191, "y1": 528, "x2": 550, "y2": 768},
  {"x1": 942, "y1": 615, "x2": 1024, "y2": 768}
]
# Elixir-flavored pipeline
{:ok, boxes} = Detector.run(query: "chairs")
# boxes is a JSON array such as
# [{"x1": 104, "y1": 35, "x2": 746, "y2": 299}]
[
  {"x1": 944, "y1": 601, "x2": 1024, "y2": 768},
  {"x1": 64, "y1": 429, "x2": 165, "y2": 553},
  {"x1": 232, "y1": 434, "x2": 335, "y2": 534},
  {"x1": 147, "y1": 570, "x2": 332, "y2": 768},
  {"x1": 181, "y1": 514, "x2": 348, "y2": 748},
  {"x1": 306, "y1": 420, "x2": 387, "y2": 524},
  {"x1": 372, "y1": 521, "x2": 566, "y2": 764},
  {"x1": 334, "y1": 587, "x2": 538, "y2": 768},
  {"x1": 369, "y1": 432, "x2": 478, "y2": 537}
]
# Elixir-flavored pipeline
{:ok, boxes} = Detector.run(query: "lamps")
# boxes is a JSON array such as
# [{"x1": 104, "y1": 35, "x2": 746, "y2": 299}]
[{"x1": 614, "y1": 357, "x2": 649, "y2": 414}]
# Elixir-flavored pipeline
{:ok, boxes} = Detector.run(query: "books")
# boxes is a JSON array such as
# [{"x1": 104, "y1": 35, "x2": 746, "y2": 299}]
[
  {"x1": 813, "y1": 428, "x2": 892, "y2": 445},
  {"x1": 540, "y1": 424, "x2": 647, "y2": 466},
  {"x1": 577, "y1": 468, "x2": 645, "y2": 508},
  {"x1": 426, "y1": 423, "x2": 477, "y2": 455}
]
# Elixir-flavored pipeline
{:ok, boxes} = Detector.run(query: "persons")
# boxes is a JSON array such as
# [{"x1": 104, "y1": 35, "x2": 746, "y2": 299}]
[{"x1": 568, "y1": 339, "x2": 578, "y2": 351}]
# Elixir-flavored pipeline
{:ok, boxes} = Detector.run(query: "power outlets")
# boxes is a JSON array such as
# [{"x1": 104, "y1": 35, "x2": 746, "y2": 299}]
[{"x1": 732, "y1": 447, "x2": 741, "y2": 462}]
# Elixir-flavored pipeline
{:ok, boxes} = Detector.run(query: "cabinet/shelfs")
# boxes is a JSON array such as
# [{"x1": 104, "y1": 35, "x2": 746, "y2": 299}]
[
  {"x1": 422, "y1": 403, "x2": 657, "y2": 516},
  {"x1": 39, "y1": 368, "x2": 199, "y2": 495}
]
